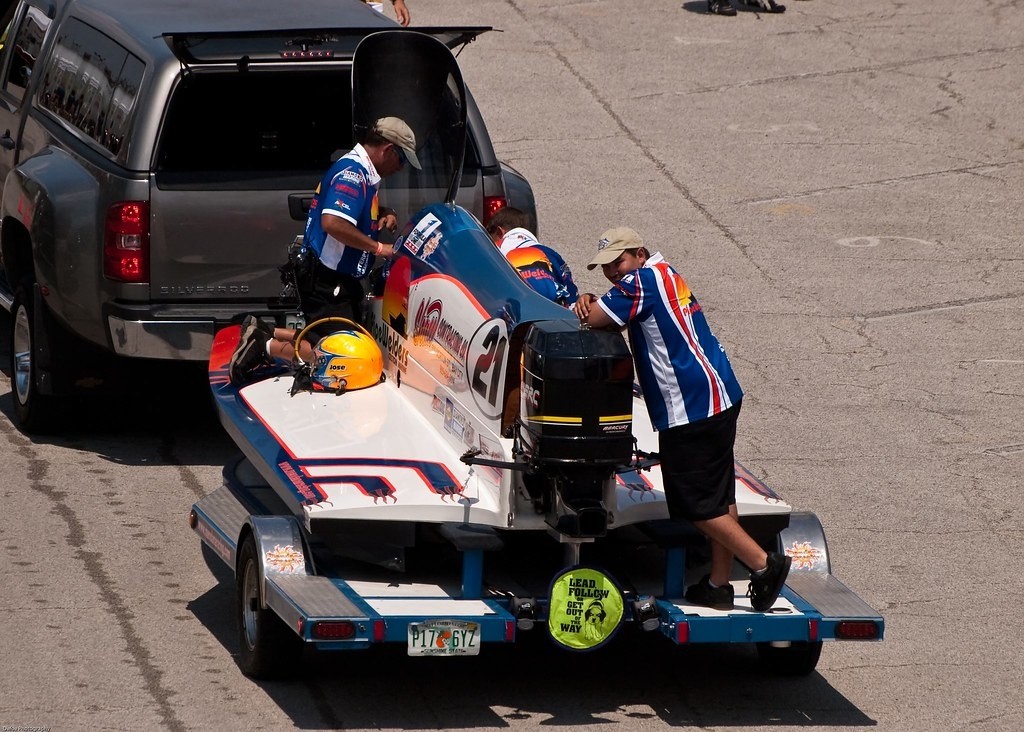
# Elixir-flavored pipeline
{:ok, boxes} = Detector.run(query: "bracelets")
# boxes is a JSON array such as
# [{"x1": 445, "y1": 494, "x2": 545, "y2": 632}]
[
  {"x1": 373, "y1": 240, "x2": 384, "y2": 256},
  {"x1": 383, "y1": 208, "x2": 398, "y2": 215}
]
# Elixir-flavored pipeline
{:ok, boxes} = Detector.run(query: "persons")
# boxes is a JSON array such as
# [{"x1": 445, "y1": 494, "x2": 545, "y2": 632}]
[
  {"x1": 487, "y1": 206, "x2": 581, "y2": 311},
  {"x1": 359, "y1": 0, "x2": 411, "y2": 27},
  {"x1": 572, "y1": 225, "x2": 794, "y2": 613},
  {"x1": 276, "y1": 115, "x2": 424, "y2": 339}
]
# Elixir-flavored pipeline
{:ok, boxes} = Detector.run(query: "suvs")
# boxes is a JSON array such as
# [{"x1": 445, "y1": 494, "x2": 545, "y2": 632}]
[{"x1": 0, "y1": 0, "x2": 541, "y2": 431}]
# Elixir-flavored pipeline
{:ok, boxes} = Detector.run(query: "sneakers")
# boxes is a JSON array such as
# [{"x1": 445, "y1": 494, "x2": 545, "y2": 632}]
[
  {"x1": 684, "y1": 574, "x2": 735, "y2": 610},
  {"x1": 240, "y1": 315, "x2": 276, "y2": 337},
  {"x1": 746, "y1": 552, "x2": 792, "y2": 612},
  {"x1": 228, "y1": 325, "x2": 272, "y2": 388}
]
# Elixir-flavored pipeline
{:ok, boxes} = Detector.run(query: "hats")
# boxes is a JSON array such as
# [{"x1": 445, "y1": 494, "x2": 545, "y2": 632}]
[
  {"x1": 369, "y1": 116, "x2": 423, "y2": 171},
  {"x1": 587, "y1": 227, "x2": 643, "y2": 271}
]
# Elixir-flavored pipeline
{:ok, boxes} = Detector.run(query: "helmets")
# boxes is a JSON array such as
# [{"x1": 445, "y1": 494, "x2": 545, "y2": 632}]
[{"x1": 311, "y1": 329, "x2": 386, "y2": 394}]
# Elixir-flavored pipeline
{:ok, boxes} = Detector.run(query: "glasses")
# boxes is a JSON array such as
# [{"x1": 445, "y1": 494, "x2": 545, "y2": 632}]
[{"x1": 395, "y1": 146, "x2": 407, "y2": 166}]
